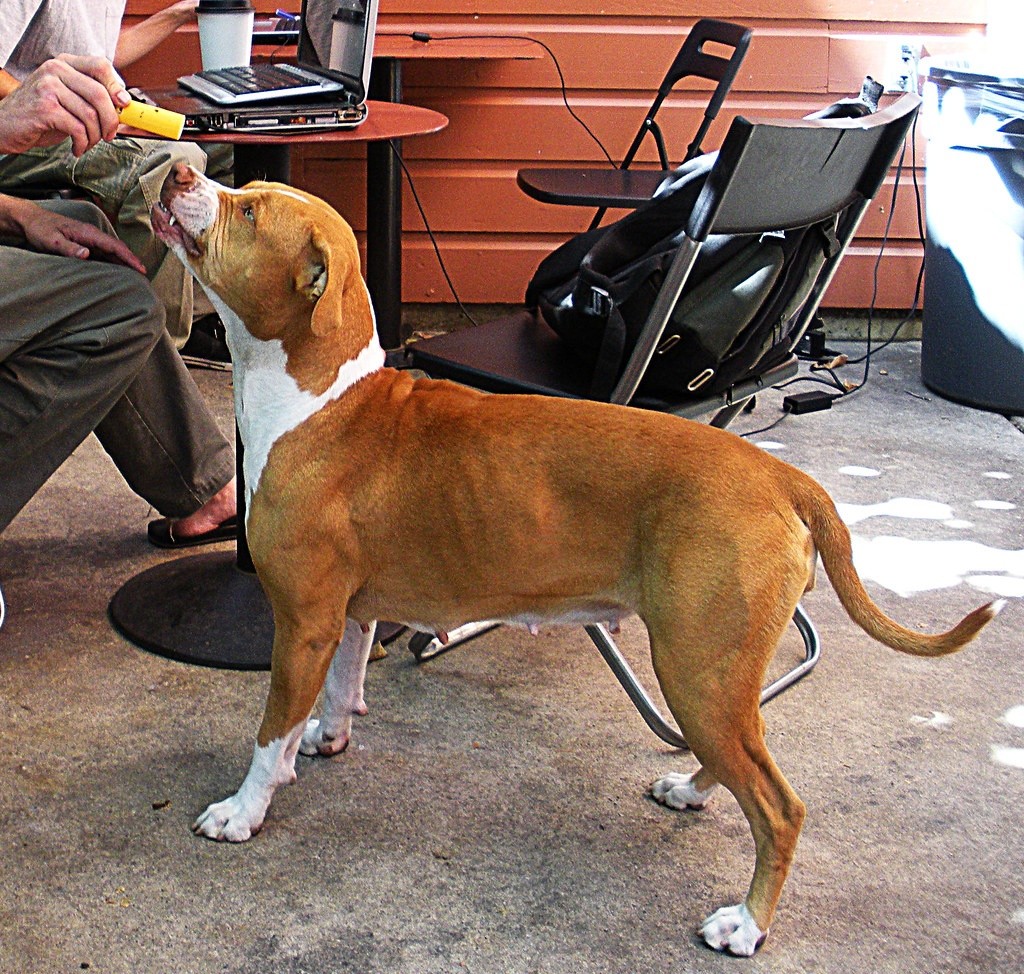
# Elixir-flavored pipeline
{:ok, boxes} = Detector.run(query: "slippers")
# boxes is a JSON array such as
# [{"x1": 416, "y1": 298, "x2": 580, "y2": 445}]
[{"x1": 148, "y1": 514, "x2": 237, "y2": 549}]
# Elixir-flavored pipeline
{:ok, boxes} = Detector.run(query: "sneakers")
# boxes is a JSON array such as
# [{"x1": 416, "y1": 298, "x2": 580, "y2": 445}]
[{"x1": 176, "y1": 328, "x2": 233, "y2": 374}]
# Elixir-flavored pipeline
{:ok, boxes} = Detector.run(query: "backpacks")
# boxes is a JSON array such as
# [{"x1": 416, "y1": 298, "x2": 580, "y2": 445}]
[{"x1": 535, "y1": 74, "x2": 886, "y2": 402}]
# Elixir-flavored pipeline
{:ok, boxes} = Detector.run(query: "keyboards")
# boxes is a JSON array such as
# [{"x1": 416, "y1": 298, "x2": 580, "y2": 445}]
[{"x1": 176, "y1": 63, "x2": 344, "y2": 108}]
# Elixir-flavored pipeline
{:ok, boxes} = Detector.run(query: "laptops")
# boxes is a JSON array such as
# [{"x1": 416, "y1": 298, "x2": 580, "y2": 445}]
[{"x1": 126, "y1": 0, "x2": 379, "y2": 132}]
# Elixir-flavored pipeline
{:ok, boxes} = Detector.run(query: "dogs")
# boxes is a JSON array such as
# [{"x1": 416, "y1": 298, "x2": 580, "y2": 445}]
[{"x1": 150, "y1": 162, "x2": 1008, "y2": 958}]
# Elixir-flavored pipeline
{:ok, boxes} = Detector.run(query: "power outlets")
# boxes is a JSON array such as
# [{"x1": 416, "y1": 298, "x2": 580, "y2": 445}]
[{"x1": 883, "y1": 39, "x2": 918, "y2": 96}]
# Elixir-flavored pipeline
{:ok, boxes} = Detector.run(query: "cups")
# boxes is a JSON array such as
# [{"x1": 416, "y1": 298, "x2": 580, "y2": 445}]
[{"x1": 194, "y1": 0, "x2": 256, "y2": 71}]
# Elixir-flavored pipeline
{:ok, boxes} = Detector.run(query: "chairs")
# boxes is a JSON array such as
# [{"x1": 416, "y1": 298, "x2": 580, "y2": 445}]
[
  {"x1": 403, "y1": 94, "x2": 927, "y2": 751},
  {"x1": 516, "y1": 19, "x2": 752, "y2": 234}
]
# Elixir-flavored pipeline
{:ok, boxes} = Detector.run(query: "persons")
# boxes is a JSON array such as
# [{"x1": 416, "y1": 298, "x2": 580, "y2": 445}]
[
  {"x1": 0, "y1": 0, "x2": 244, "y2": 369},
  {"x1": 0, "y1": 55, "x2": 244, "y2": 636}
]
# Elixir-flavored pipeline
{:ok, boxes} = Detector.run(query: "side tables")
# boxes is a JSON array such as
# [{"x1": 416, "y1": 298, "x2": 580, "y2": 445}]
[
  {"x1": 108, "y1": 95, "x2": 450, "y2": 671},
  {"x1": 250, "y1": 17, "x2": 548, "y2": 380}
]
